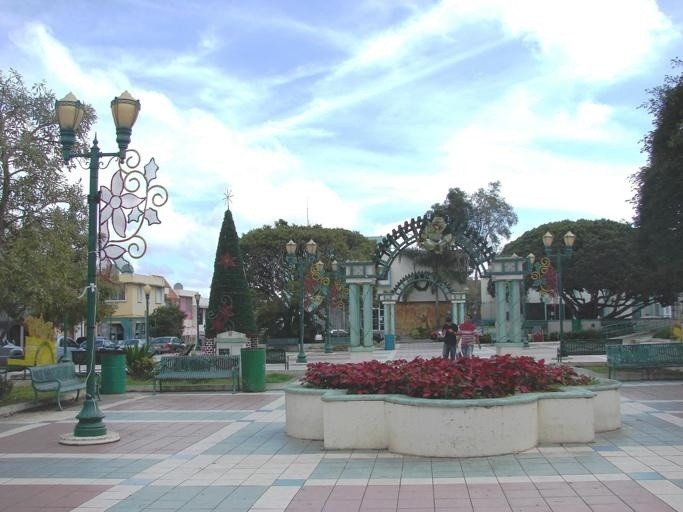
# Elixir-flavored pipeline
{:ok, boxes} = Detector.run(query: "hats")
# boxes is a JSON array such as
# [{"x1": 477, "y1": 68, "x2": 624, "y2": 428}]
[{"x1": 1, "y1": 336, "x2": 187, "y2": 372}]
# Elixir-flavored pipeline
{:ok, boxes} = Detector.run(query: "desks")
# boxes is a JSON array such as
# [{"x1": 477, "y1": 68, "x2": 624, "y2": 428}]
[
  {"x1": 142, "y1": 282, "x2": 152, "y2": 348},
  {"x1": 54, "y1": 90, "x2": 141, "y2": 446},
  {"x1": 543, "y1": 231, "x2": 576, "y2": 359},
  {"x1": 286, "y1": 238, "x2": 339, "y2": 363},
  {"x1": 511, "y1": 252, "x2": 535, "y2": 348},
  {"x1": 194, "y1": 291, "x2": 201, "y2": 351}
]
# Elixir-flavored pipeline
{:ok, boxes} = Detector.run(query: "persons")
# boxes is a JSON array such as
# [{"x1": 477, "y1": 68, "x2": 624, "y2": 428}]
[{"x1": 442, "y1": 314, "x2": 481, "y2": 360}]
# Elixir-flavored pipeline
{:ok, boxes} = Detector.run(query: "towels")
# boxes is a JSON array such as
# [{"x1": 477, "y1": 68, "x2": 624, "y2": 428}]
[
  {"x1": 384, "y1": 335, "x2": 395, "y2": 349},
  {"x1": 241, "y1": 348, "x2": 265, "y2": 393},
  {"x1": 99, "y1": 349, "x2": 127, "y2": 394}
]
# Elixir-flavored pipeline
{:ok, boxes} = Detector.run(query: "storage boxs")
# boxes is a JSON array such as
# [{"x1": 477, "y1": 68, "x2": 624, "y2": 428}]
[
  {"x1": 265, "y1": 348, "x2": 289, "y2": 370},
  {"x1": 556, "y1": 338, "x2": 623, "y2": 361},
  {"x1": 605, "y1": 342, "x2": 682, "y2": 381},
  {"x1": 151, "y1": 355, "x2": 240, "y2": 394},
  {"x1": 23, "y1": 360, "x2": 101, "y2": 411}
]
